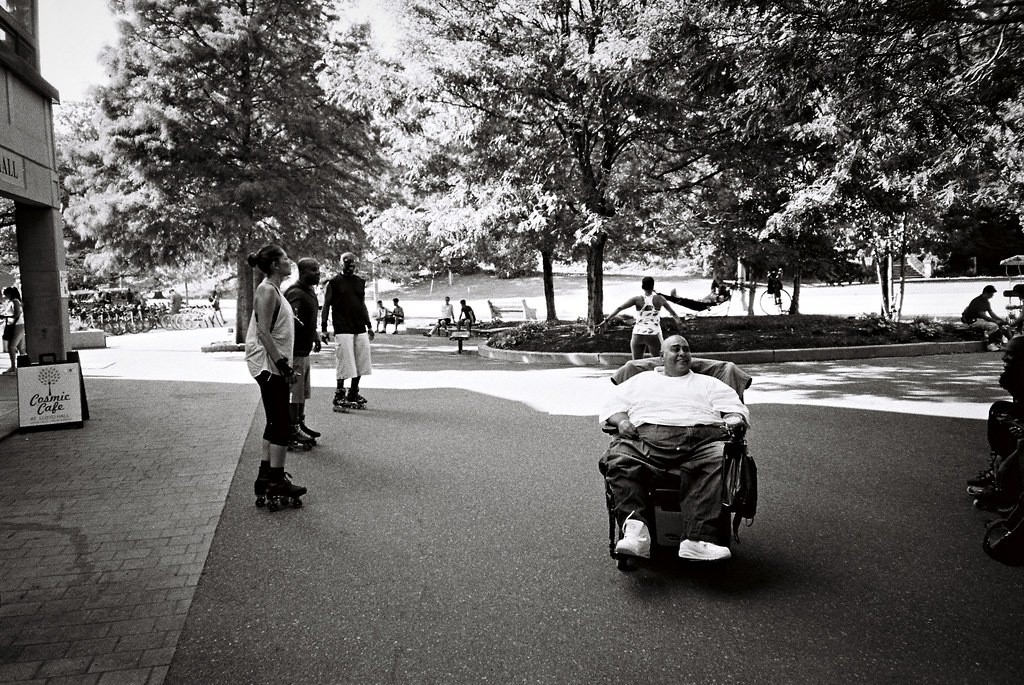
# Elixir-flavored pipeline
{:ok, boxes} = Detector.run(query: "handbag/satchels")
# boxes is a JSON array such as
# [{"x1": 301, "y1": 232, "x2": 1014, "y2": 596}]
[
  {"x1": 2, "y1": 317, "x2": 15, "y2": 340},
  {"x1": 718, "y1": 440, "x2": 759, "y2": 519}
]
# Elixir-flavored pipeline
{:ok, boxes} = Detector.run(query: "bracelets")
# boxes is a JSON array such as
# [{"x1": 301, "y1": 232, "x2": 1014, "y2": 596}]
[{"x1": 618, "y1": 420, "x2": 630, "y2": 426}]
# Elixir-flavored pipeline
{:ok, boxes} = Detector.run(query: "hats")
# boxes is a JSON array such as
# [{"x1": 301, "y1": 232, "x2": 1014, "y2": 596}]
[{"x1": 983, "y1": 285, "x2": 997, "y2": 294}]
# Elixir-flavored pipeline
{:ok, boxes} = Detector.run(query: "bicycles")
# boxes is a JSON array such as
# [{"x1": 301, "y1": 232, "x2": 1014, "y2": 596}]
[
  {"x1": 759, "y1": 268, "x2": 792, "y2": 316},
  {"x1": 68, "y1": 300, "x2": 226, "y2": 337}
]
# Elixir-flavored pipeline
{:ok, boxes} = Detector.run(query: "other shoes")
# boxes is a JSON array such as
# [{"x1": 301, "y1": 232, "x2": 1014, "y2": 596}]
[{"x1": 987, "y1": 343, "x2": 1000, "y2": 351}]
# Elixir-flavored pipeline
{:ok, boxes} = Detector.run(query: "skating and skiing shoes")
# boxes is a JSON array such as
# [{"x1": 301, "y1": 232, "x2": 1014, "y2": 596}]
[
  {"x1": 332, "y1": 388, "x2": 352, "y2": 413},
  {"x1": 253, "y1": 471, "x2": 308, "y2": 511},
  {"x1": 288, "y1": 419, "x2": 321, "y2": 452},
  {"x1": 346, "y1": 388, "x2": 367, "y2": 410}
]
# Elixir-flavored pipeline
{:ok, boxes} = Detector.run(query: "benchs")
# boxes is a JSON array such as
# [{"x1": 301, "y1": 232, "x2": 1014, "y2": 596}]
[
  {"x1": 429, "y1": 320, "x2": 517, "y2": 355},
  {"x1": 488, "y1": 299, "x2": 537, "y2": 320}
]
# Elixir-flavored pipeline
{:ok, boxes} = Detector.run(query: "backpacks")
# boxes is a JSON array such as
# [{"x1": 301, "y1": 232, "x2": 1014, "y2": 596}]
[{"x1": 962, "y1": 306, "x2": 977, "y2": 324}]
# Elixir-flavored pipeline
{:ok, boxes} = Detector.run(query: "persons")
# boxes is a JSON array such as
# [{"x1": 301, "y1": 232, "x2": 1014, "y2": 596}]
[
  {"x1": 320, "y1": 251, "x2": 376, "y2": 412},
  {"x1": 210, "y1": 285, "x2": 228, "y2": 327},
  {"x1": 168, "y1": 289, "x2": 183, "y2": 314},
  {"x1": 283, "y1": 258, "x2": 320, "y2": 449},
  {"x1": 598, "y1": 335, "x2": 754, "y2": 557},
  {"x1": 426, "y1": 297, "x2": 457, "y2": 337},
  {"x1": 457, "y1": 299, "x2": 476, "y2": 332},
  {"x1": 98, "y1": 287, "x2": 143, "y2": 312},
  {"x1": 379, "y1": 298, "x2": 404, "y2": 334},
  {"x1": 375, "y1": 300, "x2": 389, "y2": 333},
  {"x1": 0, "y1": 287, "x2": 26, "y2": 373},
  {"x1": 595, "y1": 277, "x2": 688, "y2": 357},
  {"x1": 964, "y1": 284, "x2": 1024, "y2": 509},
  {"x1": 247, "y1": 245, "x2": 309, "y2": 511}
]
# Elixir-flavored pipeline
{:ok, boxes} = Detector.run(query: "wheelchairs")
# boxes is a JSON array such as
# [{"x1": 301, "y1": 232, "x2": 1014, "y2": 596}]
[
  {"x1": 605, "y1": 424, "x2": 759, "y2": 575},
  {"x1": 976, "y1": 411, "x2": 1024, "y2": 569}
]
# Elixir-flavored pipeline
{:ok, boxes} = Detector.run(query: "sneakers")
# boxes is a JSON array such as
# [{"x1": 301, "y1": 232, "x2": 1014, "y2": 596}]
[
  {"x1": 615, "y1": 510, "x2": 652, "y2": 560},
  {"x1": 679, "y1": 539, "x2": 731, "y2": 560}
]
